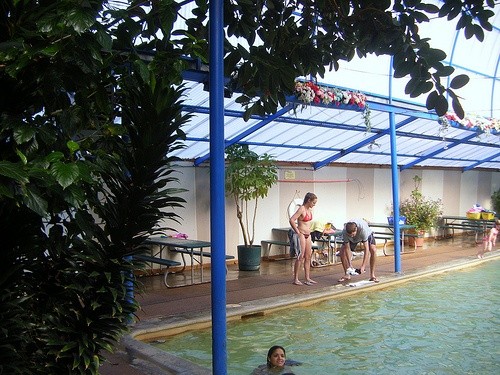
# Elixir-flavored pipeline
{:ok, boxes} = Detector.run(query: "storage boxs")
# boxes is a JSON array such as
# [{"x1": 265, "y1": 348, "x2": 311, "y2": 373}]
[
  {"x1": 465, "y1": 212, "x2": 480, "y2": 219},
  {"x1": 388, "y1": 216, "x2": 406, "y2": 225},
  {"x1": 481, "y1": 211, "x2": 495, "y2": 220}
]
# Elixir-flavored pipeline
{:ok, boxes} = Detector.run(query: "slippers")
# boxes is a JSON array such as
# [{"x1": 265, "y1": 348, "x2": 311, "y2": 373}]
[
  {"x1": 338, "y1": 277, "x2": 346, "y2": 282},
  {"x1": 369, "y1": 277, "x2": 380, "y2": 282}
]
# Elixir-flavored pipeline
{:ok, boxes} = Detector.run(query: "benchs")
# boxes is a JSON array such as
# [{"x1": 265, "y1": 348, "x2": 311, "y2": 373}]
[
  {"x1": 373, "y1": 231, "x2": 417, "y2": 255},
  {"x1": 170, "y1": 249, "x2": 234, "y2": 283},
  {"x1": 134, "y1": 254, "x2": 181, "y2": 288},
  {"x1": 314, "y1": 238, "x2": 354, "y2": 265},
  {"x1": 441, "y1": 222, "x2": 496, "y2": 243},
  {"x1": 262, "y1": 238, "x2": 318, "y2": 264}
]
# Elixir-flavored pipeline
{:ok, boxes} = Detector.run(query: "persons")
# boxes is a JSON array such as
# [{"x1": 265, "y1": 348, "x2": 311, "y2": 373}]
[
  {"x1": 338, "y1": 218, "x2": 380, "y2": 284},
  {"x1": 290, "y1": 191, "x2": 319, "y2": 286},
  {"x1": 475, "y1": 219, "x2": 500, "y2": 259},
  {"x1": 250, "y1": 345, "x2": 294, "y2": 375}
]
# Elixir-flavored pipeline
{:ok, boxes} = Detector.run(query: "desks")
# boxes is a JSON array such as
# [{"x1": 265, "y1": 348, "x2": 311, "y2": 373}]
[
  {"x1": 441, "y1": 215, "x2": 495, "y2": 243},
  {"x1": 368, "y1": 222, "x2": 417, "y2": 255},
  {"x1": 273, "y1": 226, "x2": 345, "y2": 267},
  {"x1": 144, "y1": 237, "x2": 228, "y2": 288}
]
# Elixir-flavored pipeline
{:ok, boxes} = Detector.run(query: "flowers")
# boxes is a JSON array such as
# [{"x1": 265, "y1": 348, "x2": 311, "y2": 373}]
[
  {"x1": 295, "y1": 79, "x2": 371, "y2": 136},
  {"x1": 438, "y1": 111, "x2": 500, "y2": 150}
]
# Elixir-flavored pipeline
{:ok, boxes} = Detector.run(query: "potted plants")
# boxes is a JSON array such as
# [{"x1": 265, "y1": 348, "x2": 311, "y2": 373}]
[
  {"x1": 224, "y1": 142, "x2": 278, "y2": 272},
  {"x1": 400, "y1": 194, "x2": 444, "y2": 246}
]
